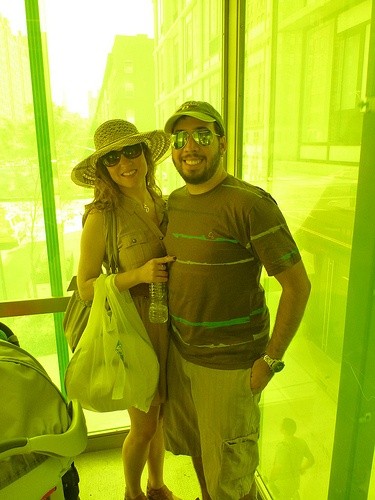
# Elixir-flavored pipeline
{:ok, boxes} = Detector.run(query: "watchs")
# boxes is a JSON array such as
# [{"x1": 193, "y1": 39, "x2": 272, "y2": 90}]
[{"x1": 261, "y1": 353, "x2": 285, "y2": 372}]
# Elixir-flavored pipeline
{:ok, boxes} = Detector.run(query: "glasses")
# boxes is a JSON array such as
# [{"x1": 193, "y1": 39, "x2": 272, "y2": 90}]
[
  {"x1": 102, "y1": 144, "x2": 143, "y2": 167},
  {"x1": 171, "y1": 128, "x2": 221, "y2": 150}
]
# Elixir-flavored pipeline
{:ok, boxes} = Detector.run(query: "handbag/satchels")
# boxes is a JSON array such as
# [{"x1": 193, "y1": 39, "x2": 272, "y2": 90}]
[
  {"x1": 62, "y1": 276, "x2": 111, "y2": 354},
  {"x1": 64, "y1": 274, "x2": 160, "y2": 416}
]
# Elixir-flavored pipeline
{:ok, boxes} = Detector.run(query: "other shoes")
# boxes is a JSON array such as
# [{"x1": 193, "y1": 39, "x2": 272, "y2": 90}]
[
  {"x1": 123, "y1": 487, "x2": 148, "y2": 500},
  {"x1": 147, "y1": 480, "x2": 174, "y2": 500}
]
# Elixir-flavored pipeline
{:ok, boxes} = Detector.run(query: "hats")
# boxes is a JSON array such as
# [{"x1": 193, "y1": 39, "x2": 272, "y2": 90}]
[
  {"x1": 71, "y1": 118, "x2": 171, "y2": 188},
  {"x1": 164, "y1": 100, "x2": 226, "y2": 136}
]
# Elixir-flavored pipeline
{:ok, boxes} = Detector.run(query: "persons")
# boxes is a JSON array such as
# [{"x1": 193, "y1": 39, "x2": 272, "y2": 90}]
[
  {"x1": 162, "y1": 100, "x2": 311, "y2": 500},
  {"x1": 273, "y1": 418, "x2": 315, "y2": 500},
  {"x1": 76, "y1": 119, "x2": 183, "y2": 500}
]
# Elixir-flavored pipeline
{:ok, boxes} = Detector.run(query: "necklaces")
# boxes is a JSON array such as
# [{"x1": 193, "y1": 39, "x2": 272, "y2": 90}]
[{"x1": 137, "y1": 193, "x2": 151, "y2": 213}]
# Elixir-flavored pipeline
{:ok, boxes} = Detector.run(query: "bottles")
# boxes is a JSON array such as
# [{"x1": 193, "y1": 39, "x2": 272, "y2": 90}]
[{"x1": 148, "y1": 282, "x2": 169, "y2": 324}]
